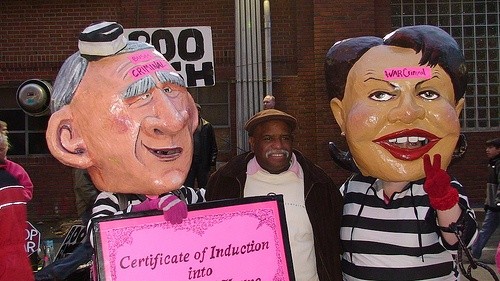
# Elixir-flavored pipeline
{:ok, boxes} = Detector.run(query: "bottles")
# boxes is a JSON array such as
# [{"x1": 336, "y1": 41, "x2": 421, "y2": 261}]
[{"x1": 42, "y1": 241, "x2": 55, "y2": 266}]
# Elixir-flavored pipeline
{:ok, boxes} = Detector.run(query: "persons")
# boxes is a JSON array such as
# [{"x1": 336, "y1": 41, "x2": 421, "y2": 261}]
[
  {"x1": 184, "y1": 102, "x2": 218, "y2": 189},
  {"x1": 471, "y1": 138, "x2": 500, "y2": 261},
  {"x1": 248, "y1": 96, "x2": 276, "y2": 110},
  {"x1": 35, "y1": 169, "x2": 98, "y2": 281},
  {"x1": 46, "y1": 22, "x2": 206, "y2": 281},
  {"x1": 206, "y1": 109, "x2": 344, "y2": 281},
  {"x1": 325, "y1": 25, "x2": 479, "y2": 281},
  {"x1": 0, "y1": 120, "x2": 36, "y2": 281}
]
